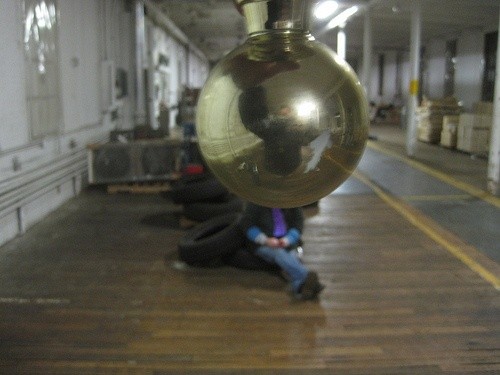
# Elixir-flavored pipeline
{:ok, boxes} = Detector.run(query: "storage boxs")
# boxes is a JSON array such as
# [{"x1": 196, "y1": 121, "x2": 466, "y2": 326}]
[{"x1": 417, "y1": 94, "x2": 494, "y2": 151}]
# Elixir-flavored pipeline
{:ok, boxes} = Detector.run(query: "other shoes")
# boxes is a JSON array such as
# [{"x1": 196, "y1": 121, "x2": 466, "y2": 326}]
[
  {"x1": 303, "y1": 272, "x2": 317, "y2": 299},
  {"x1": 314, "y1": 284, "x2": 325, "y2": 298}
]
